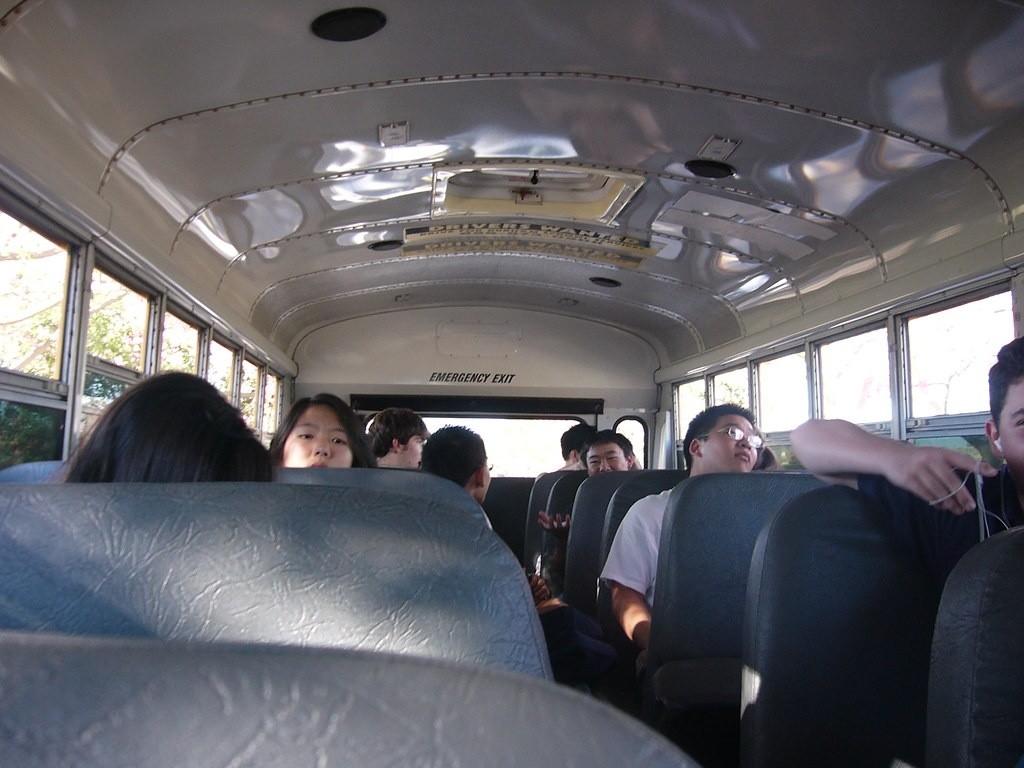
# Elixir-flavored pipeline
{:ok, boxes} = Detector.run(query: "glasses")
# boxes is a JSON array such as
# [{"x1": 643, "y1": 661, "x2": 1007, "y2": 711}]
[
  {"x1": 697, "y1": 426, "x2": 763, "y2": 448},
  {"x1": 483, "y1": 462, "x2": 493, "y2": 471}
]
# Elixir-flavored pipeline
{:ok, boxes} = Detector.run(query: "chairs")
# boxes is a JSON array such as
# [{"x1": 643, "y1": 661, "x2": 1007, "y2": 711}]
[{"x1": 0, "y1": 460, "x2": 1024, "y2": 768}]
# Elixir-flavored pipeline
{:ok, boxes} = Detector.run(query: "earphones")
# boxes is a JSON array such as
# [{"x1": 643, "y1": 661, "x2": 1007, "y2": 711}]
[{"x1": 994, "y1": 435, "x2": 1003, "y2": 456}]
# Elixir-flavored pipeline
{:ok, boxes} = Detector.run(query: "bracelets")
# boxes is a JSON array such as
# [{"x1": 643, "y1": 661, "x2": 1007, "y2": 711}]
[{"x1": 545, "y1": 589, "x2": 552, "y2": 600}]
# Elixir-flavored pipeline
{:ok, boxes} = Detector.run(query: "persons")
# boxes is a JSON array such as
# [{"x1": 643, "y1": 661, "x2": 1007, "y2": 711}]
[
  {"x1": 790, "y1": 335, "x2": 1024, "y2": 599},
  {"x1": 268, "y1": 392, "x2": 379, "y2": 469},
  {"x1": 366, "y1": 406, "x2": 426, "y2": 470},
  {"x1": 57, "y1": 372, "x2": 279, "y2": 486},
  {"x1": 537, "y1": 430, "x2": 635, "y2": 547},
  {"x1": 561, "y1": 423, "x2": 596, "y2": 470},
  {"x1": 599, "y1": 405, "x2": 777, "y2": 649},
  {"x1": 420, "y1": 426, "x2": 629, "y2": 707}
]
